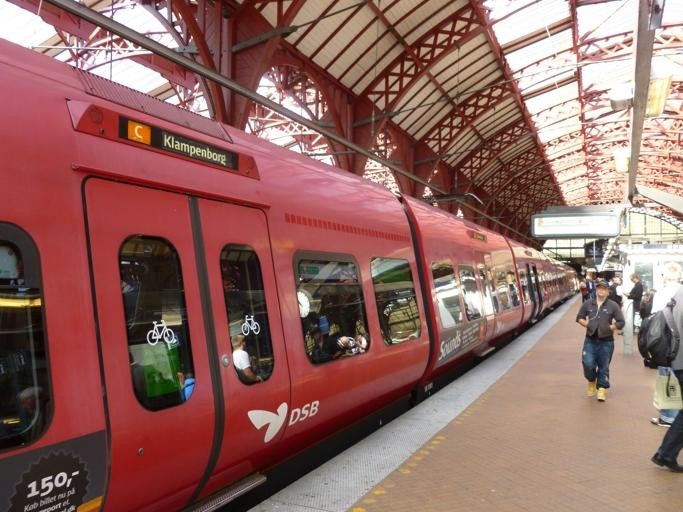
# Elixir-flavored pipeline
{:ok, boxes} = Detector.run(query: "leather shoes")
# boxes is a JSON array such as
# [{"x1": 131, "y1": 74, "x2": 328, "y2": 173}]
[{"x1": 650, "y1": 453, "x2": 683, "y2": 472}]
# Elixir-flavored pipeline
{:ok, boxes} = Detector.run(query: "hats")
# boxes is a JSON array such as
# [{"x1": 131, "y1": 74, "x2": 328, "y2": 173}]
[{"x1": 595, "y1": 280, "x2": 609, "y2": 288}]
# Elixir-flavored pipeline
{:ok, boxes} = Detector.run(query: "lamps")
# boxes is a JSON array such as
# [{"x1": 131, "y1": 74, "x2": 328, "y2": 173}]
[{"x1": 610, "y1": 84, "x2": 634, "y2": 111}]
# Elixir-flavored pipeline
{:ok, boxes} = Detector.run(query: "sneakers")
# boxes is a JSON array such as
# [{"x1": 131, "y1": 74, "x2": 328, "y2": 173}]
[
  {"x1": 649, "y1": 416, "x2": 672, "y2": 427},
  {"x1": 597, "y1": 387, "x2": 606, "y2": 401},
  {"x1": 585, "y1": 379, "x2": 595, "y2": 396}
]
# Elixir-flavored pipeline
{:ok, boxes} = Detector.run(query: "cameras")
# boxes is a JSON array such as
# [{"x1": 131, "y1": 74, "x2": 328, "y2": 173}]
[{"x1": 644, "y1": 359, "x2": 658, "y2": 370}]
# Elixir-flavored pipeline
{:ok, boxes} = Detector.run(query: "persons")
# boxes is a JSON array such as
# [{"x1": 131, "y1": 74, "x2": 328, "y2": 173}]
[
  {"x1": 15, "y1": 270, "x2": 540, "y2": 441},
  {"x1": 575, "y1": 280, "x2": 625, "y2": 402},
  {"x1": 648, "y1": 260, "x2": 682, "y2": 427},
  {"x1": 577, "y1": 273, "x2": 658, "y2": 336},
  {"x1": 648, "y1": 286, "x2": 682, "y2": 474}
]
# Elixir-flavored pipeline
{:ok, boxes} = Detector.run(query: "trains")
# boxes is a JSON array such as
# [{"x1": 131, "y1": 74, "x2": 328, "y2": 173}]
[{"x1": 1, "y1": 39, "x2": 580, "y2": 511}]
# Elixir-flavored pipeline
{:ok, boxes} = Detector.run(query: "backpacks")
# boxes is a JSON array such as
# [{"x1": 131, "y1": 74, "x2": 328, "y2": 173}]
[{"x1": 637, "y1": 304, "x2": 679, "y2": 368}]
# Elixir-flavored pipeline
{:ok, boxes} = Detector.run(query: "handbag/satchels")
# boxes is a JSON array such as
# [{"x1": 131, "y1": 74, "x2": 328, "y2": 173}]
[{"x1": 653, "y1": 374, "x2": 682, "y2": 410}]
